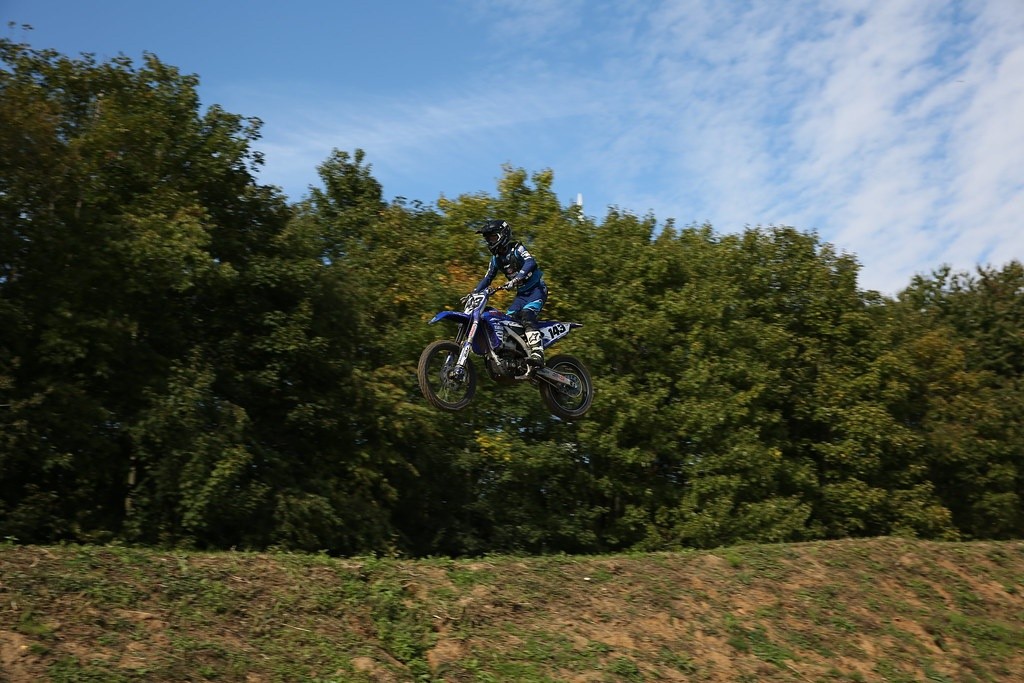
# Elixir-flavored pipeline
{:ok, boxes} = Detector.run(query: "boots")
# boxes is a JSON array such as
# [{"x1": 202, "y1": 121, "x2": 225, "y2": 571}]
[{"x1": 525, "y1": 329, "x2": 544, "y2": 365}]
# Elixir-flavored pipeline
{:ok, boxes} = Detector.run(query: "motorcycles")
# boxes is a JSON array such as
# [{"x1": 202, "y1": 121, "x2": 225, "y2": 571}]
[{"x1": 417, "y1": 282, "x2": 593, "y2": 420}]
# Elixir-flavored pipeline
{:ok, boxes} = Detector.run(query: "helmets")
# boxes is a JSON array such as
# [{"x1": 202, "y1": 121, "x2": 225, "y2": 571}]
[{"x1": 475, "y1": 219, "x2": 513, "y2": 255}]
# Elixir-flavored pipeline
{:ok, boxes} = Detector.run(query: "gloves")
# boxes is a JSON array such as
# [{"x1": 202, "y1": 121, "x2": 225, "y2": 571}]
[
  {"x1": 460, "y1": 295, "x2": 469, "y2": 304},
  {"x1": 504, "y1": 277, "x2": 520, "y2": 292}
]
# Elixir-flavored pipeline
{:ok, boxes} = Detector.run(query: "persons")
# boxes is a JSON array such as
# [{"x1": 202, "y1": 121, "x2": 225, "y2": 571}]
[{"x1": 472, "y1": 219, "x2": 547, "y2": 368}]
